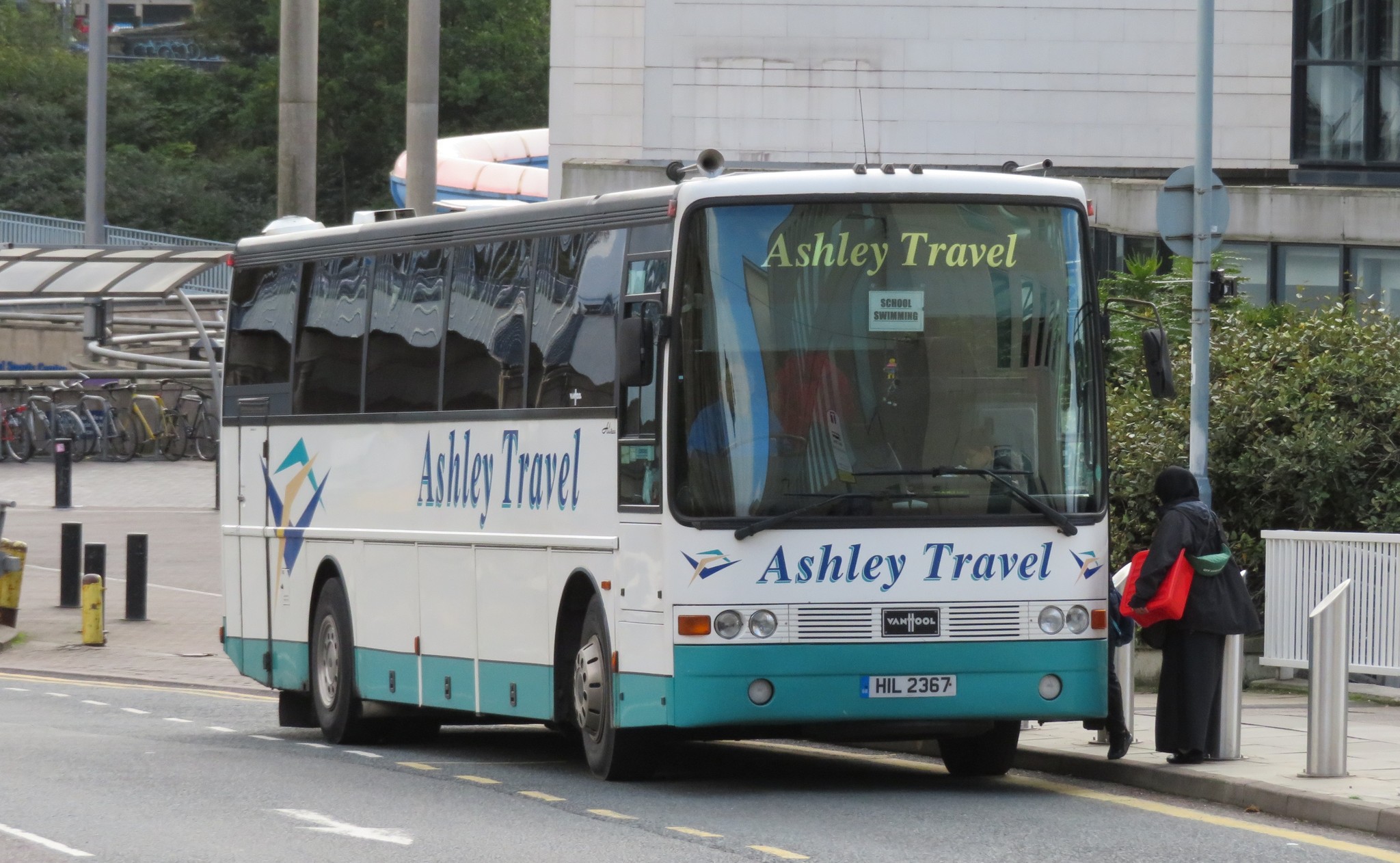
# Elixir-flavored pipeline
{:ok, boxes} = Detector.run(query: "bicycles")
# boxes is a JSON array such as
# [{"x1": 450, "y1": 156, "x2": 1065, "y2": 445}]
[
  {"x1": 0, "y1": 371, "x2": 188, "y2": 464},
  {"x1": 155, "y1": 378, "x2": 219, "y2": 462}
]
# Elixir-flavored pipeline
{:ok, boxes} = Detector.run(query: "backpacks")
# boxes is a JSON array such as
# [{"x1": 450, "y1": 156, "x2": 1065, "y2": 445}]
[{"x1": 1108, "y1": 588, "x2": 1134, "y2": 647}]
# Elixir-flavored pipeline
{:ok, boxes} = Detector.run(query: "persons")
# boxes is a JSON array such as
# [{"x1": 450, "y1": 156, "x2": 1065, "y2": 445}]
[
  {"x1": 686, "y1": 343, "x2": 858, "y2": 517},
  {"x1": 1128, "y1": 465, "x2": 1262, "y2": 764},
  {"x1": 1083, "y1": 570, "x2": 1134, "y2": 760}
]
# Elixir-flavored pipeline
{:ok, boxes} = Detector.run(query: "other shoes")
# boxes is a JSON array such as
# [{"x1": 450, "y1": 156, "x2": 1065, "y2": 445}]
[
  {"x1": 1166, "y1": 752, "x2": 1204, "y2": 764},
  {"x1": 1107, "y1": 727, "x2": 1133, "y2": 760}
]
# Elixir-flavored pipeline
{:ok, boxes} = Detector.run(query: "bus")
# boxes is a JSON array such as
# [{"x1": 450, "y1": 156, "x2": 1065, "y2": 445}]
[{"x1": 216, "y1": 142, "x2": 1181, "y2": 781}]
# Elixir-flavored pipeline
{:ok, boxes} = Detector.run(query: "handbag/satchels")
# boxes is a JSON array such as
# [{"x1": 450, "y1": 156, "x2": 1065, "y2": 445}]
[{"x1": 1193, "y1": 542, "x2": 1232, "y2": 577}]
